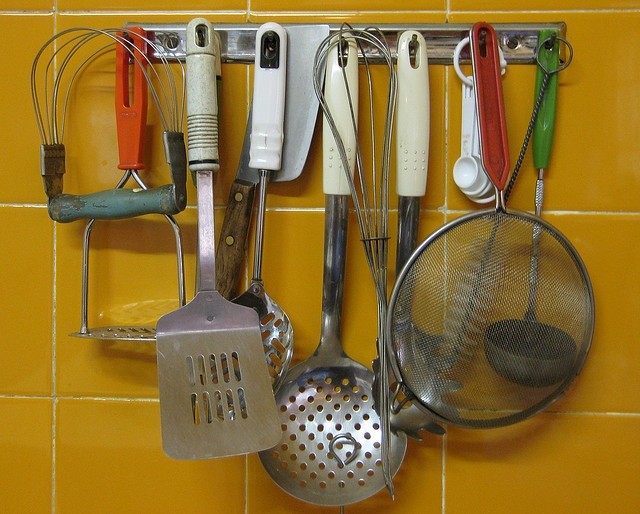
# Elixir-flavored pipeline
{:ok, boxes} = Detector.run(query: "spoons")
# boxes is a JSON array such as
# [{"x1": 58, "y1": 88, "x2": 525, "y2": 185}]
[
  {"x1": 452, "y1": 71, "x2": 496, "y2": 203},
  {"x1": 483, "y1": 24, "x2": 579, "y2": 387}
]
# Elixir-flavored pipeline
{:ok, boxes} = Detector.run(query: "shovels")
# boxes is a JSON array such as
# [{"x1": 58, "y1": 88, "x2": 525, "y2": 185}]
[{"x1": 156, "y1": 18, "x2": 282, "y2": 460}]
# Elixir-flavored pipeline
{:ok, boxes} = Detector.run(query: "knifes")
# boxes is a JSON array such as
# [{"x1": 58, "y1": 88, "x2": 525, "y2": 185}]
[{"x1": 216, "y1": 23, "x2": 330, "y2": 299}]
